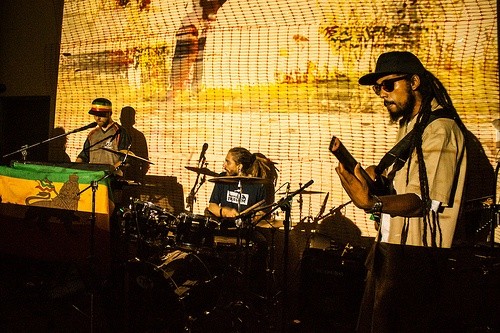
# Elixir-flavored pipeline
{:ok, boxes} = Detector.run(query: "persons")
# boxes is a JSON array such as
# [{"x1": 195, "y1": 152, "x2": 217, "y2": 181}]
[
  {"x1": 75, "y1": 98, "x2": 133, "y2": 168},
  {"x1": 208, "y1": 146, "x2": 271, "y2": 226},
  {"x1": 335, "y1": 51, "x2": 467, "y2": 333}
]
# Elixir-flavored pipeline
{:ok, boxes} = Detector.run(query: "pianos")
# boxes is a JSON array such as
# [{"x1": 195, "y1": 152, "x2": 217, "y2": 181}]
[{"x1": 8, "y1": 159, "x2": 117, "y2": 175}]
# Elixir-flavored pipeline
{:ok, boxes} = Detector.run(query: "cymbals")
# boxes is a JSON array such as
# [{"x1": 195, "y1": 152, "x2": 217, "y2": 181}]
[
  {"x1": 277, "y1": 188, "x2": 326, "y2": 195},
  {"x1": 98, "y1": 143, "x2": 155, "y2": 166},
  {"x1": 208, "y1": 170, "x2": 271, "y2": 187},
  {"x1": 255, "y1": 217, "x2": 297, "y2": 229},
  {"x1": 185, "y1": 165, "x2": 222, "y2": 179}
]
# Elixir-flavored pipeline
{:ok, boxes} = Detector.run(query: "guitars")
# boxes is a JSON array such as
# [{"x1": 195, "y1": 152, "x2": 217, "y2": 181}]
[{"x1": 329, "y1": 136, "x2": 401, "y2": 219}]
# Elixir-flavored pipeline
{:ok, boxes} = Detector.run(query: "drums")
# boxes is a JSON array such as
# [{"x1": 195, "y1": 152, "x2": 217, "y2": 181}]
[
  {"x1": 212, "y1": 233, "x2": 255, "y2": 260},
  {"x1": 173, "y1": 212, "x2": 221, "y2": 251},
  {"x1": 118, "y1": 198, "x2": 179, "y2": 247},
  {"x1": 96, "y1": 245, "x2": 221, "y2": 332}
]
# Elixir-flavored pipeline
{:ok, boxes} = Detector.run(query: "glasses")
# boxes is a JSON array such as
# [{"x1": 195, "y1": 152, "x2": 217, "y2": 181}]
[{"x1": 372, "y1": 76, "x2": 407, "y2": 95}]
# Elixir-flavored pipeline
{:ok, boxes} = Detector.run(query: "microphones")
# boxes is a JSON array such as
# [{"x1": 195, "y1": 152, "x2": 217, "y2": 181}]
[
  {"x1": 285, "y1": 183, "x2": 290, "y2": 199},
  {"x1": 110, "y1": 129, "x2": 121, "y2": 143},
  {"x1": 70, "y1": 122, "x2": 97, "y2": 134},
  {"x1": 199, "y1": 143, "x2": 208, "y2": 162}
]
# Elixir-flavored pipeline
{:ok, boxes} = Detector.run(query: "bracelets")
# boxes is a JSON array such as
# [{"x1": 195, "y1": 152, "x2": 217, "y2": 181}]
[
  {"x1": 364, "y1": 195, "x2": 383, "y2": 212},
  {"x1": 220, "y1": 207, "x2": 223, "y2": 218}
]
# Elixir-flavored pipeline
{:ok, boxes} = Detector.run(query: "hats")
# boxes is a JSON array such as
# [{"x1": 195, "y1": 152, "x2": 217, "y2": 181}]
[
  {"x1": 88, "y1": 98, "x2": 112, "y2": 116},
  {"x1": 359, "y1": 51, "x2": 423, "y2": 85}
]
